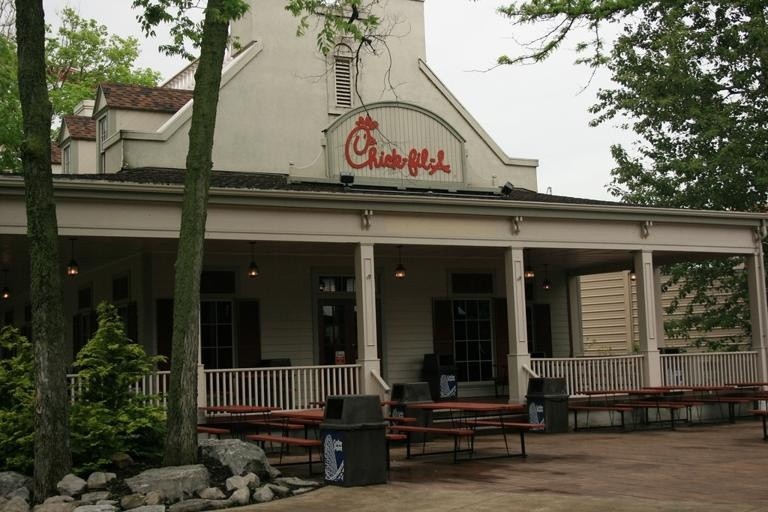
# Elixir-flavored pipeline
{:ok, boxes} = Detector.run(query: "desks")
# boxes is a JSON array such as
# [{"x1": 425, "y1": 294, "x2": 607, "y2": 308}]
[{"x1": 199, "y1": 401, "x2": 526, "y2": 463}]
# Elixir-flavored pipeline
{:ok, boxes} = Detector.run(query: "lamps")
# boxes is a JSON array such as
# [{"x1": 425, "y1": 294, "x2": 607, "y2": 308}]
[
  {"x1": 630, "y1": 251, "x2": 636, "y2": 281},
  {"x1": 247, "y1": 241, "x2": 259, "y2": 281},
  {"x1": 361, "y1": 209, "x2": 374, "y2": 226},
  {"x1": 641, "y1": 221, "x2": 653, "y2": 237},
  {"x1": 500, "y1": 180, "x2": 514, "y2": 200},
  {"x1": 339, "y1": 171, "x2": 356, "y2": 190},
  {"x1": 752, "y1": 226, "x2": 761, "y2": 242},
  {"x1": 541, "y1": 263, "x2": 551, "y2": 295},
  {"x1": 1, "y1": 268, "x2": 12, "y2": 303},
  {"x1": 510, "y1": 215, "x2": 523, "y2": 234},
  {"x1": 67, "y1": 237, "x2": 79, "y2": 278},
  {"x1": 394, "y1": 245, "x2": 407, "y2": 281},
  {"x1": 525, "y1": 248, "x2": 535, "y2": 280}
]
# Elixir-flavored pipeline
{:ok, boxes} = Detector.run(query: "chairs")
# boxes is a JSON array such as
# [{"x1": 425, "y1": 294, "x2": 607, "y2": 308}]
[{"x1": 494, "y1": 363, "x2": 508, "y2": 396}]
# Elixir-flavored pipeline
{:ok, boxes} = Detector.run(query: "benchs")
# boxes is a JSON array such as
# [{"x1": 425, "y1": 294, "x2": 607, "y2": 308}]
[
  {"x1": 197, "y1": 418, "x2": 545, "y2": 478},
  {"x1": 568, "y1": 393, "x2": 767, "y2": 440}
]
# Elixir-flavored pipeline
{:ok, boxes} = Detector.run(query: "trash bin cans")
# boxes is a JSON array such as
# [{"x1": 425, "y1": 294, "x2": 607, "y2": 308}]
[
  {"x1": 391, "y1": 353, "x2": 458, "y2": 442},
  {"x1": 318, "y1": 395, "x2": 389, "y2": 487},
  {"x1": 525, "y1": 377, "x2": 569, "y2": 434},
  {"x1": 258, "y1": 357, "x2": 292, "y2": 410},
  {"x1": 660, "y1": 348, "x2": 688, "y2": 389}
]
